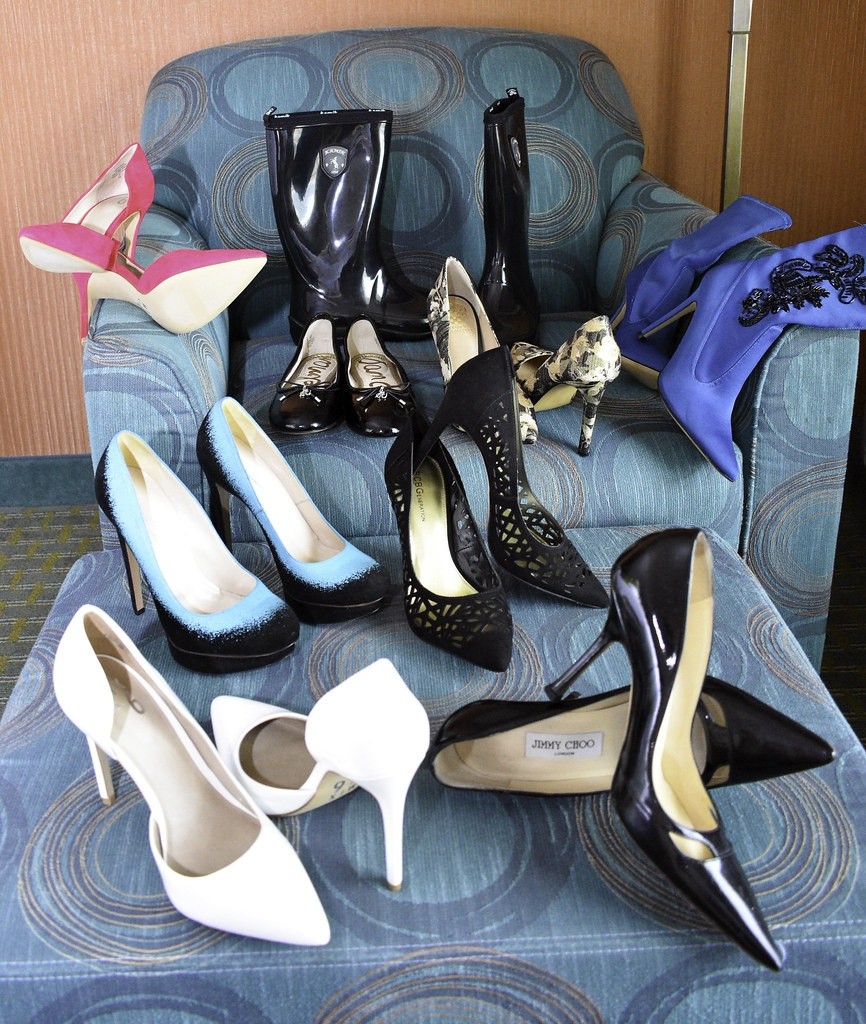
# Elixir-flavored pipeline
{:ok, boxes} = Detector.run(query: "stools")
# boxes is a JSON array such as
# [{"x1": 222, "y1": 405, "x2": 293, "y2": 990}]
[{"x1": 0, "y1": 523, "x2": 866, "y2": 1024}]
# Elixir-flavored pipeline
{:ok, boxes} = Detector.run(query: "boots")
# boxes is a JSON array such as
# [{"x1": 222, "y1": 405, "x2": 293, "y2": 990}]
[
  {"x1": 609, "y1": 194, "x2": 793, "y2": 390},
  {"x1": 478, "y1": 87, "x2": 540, "y2": 349},
  {"x1": 640, "y1": 224, "x2": 866, "y2": 483},
  {"x1": 262, "y1": 106, "x2": 431, "y2": 349}
]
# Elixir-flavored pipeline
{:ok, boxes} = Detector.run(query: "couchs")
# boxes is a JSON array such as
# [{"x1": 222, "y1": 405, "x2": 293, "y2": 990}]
[{"x1": 73, "y1": 20, "x2": 864, "y2": 671}]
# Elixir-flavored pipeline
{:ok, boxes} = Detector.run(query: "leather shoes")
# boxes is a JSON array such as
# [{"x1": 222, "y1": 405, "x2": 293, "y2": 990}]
[
  {"x1": 269, "y1": 311, "x2": 347, "y2": 435},
  {"x1": 342, "y1": 314, "x2": 418, "y2": 437}
]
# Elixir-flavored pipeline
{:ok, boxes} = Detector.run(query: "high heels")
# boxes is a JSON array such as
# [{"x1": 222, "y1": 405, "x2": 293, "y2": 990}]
[
  {"x1": 94, "y1": 430, "x2": 299, "y2": 675},
  {"x1": 384, "y1": 417, "x2": 514, "y2": 674},
  {"x1": 210, "y1": 657, "x2": 429, "y2": 892},
  {"x1": 18, "y1": 140, "x2": 155, "y2": 273},
  {"x1": 75, "y1": 248, "x2": 267, "y2": 345},
  {"x1": 432, "y1": 675, "x2": 837, "y2": 798},
  {"x1": 52, "y1": 603, "x2": 332, "y2": 948},
  {"x1": 509, "y1": 314, "x2": 621, "y2": 456},
  {"x1": 413, "y1": 344, "x2": 613, "y2": 612},
  {"x1": 427, "y1": 255, "x2": 538, "y2": 444},
  {"x1": 194, "y1": 396, "x2": 390, "y2": 625},
  {"x1": 543, "y1": 526, "x2": 784, "y2": 973}
]
